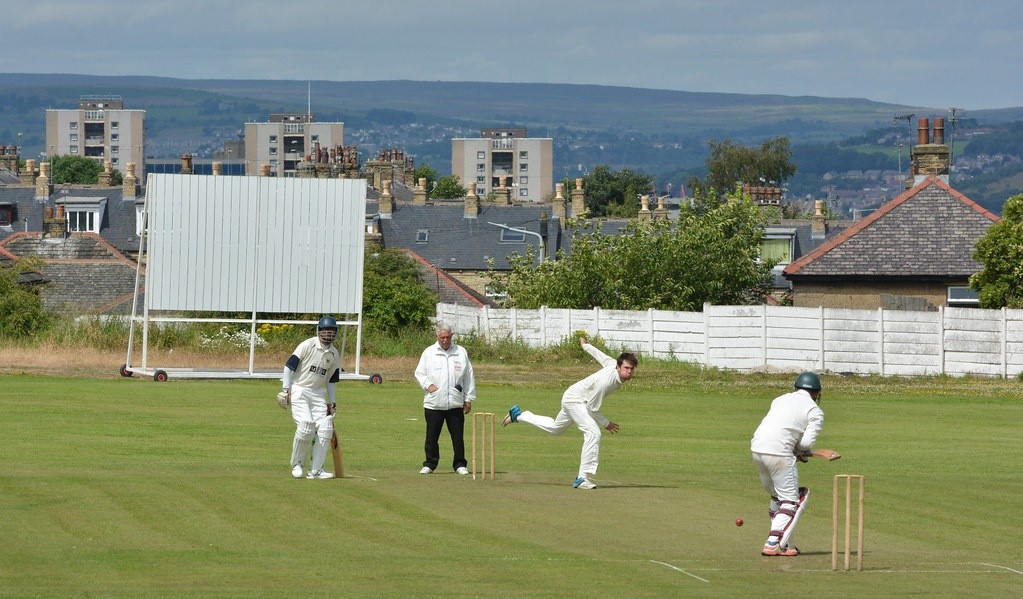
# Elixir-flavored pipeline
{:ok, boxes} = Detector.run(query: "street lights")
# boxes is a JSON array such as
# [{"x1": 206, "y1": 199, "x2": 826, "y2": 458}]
[{"x1": 487, "y1": 221, "x2": 545, "y2": 269}]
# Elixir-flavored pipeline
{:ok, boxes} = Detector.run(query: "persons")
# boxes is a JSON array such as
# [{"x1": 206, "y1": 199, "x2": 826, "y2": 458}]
[
  {"x1": 500, "y1": 337, "x2": 638, "y2": 490},
  {"x1": 750, "y1": 371, "x2": 824, "y2": 555},
  {"x1": 414, "y1": 322, "x2": 478, "y2": 475},
  {"x1": 276, "y1": 317, "x2": 340, "y2": 480}
]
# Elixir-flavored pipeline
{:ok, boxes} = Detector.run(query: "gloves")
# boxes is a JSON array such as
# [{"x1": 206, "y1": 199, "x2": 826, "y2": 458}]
[
  {"x1": 329, "y1": 402, "x2": 337, "y2": 419},
  {"x1": 277, "y1": 390, "x2": 291, "y2": 409}
]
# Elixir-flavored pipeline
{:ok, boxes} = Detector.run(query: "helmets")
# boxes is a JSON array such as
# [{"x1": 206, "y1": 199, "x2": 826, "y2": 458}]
[
  {"x1": 317, "y1": 317, "x2": 338, "y2": 335},
  {"x1": 794, "y1": 372, "x2": 822, "y2": 391}
]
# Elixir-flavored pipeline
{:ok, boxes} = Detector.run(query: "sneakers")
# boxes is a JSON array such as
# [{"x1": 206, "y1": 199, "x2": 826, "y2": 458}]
[
  {"x1": 456, "y1": 467, "x2": 469, "y2": 475},
  {"x1": 420, "y1": 466, "x2": 434, "y2": 474},
  {"x1": 502, "y1": 405, "x2": 522, "y2": 426},
  {"x1": 762, "y1": 542, "x2": 798, "y2": 556},
  {"x1": 306, "y1": 469, "x2": 334, "y2": 479},
  {"x1": 291, "y1": 465, "x2": 304, "y2": 477},
  {"x1": 572, "y1": 476, "x2": 596, "y2": 490}
]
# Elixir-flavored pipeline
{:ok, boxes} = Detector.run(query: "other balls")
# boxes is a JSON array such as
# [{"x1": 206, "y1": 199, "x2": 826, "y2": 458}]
[{"x1": 735, "y1": 519, "x2": 744, "y2": 527}]
